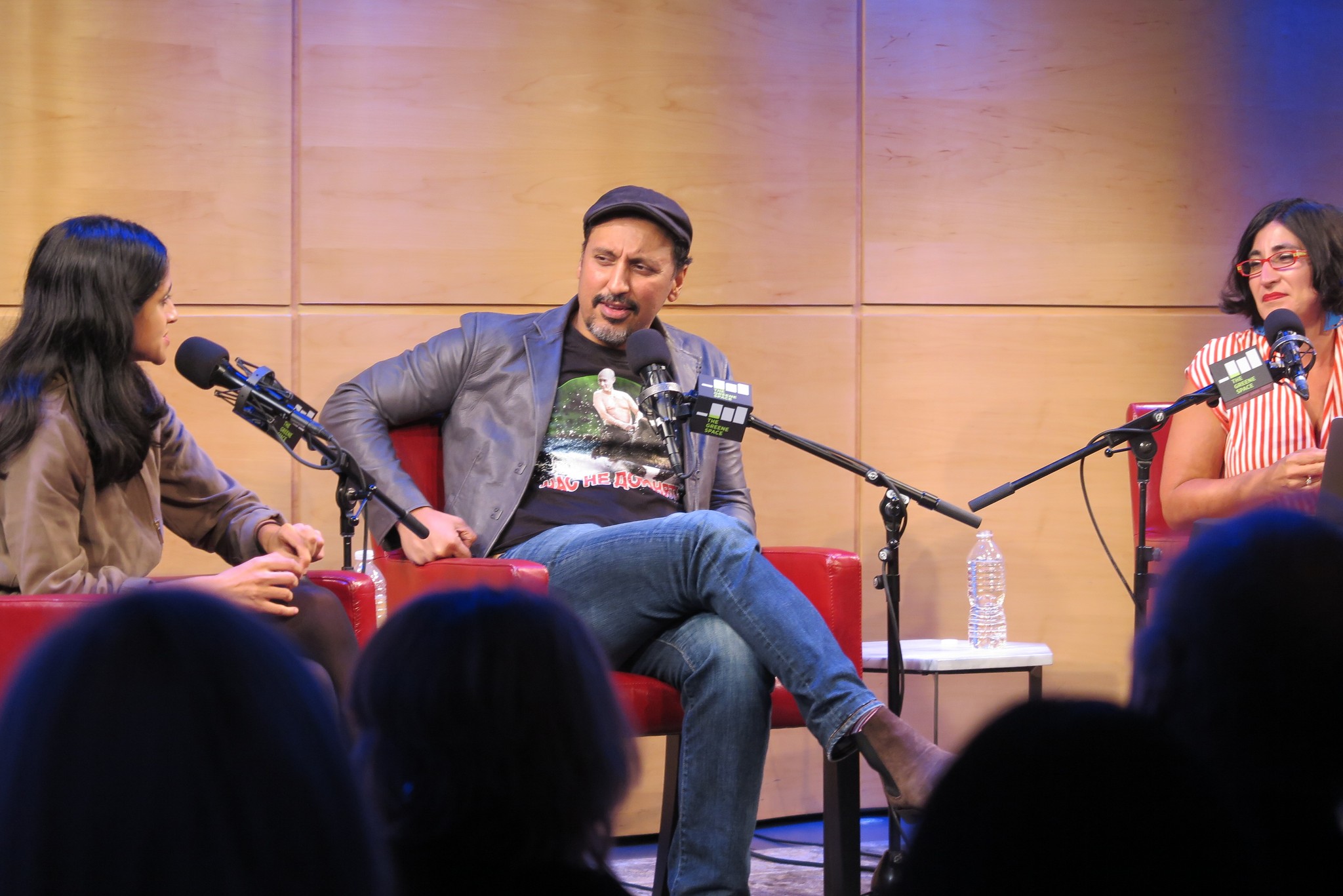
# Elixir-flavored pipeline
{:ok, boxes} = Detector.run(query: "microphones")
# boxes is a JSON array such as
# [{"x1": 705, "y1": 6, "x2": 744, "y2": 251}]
[
  {"x1": 625, "y1": 329, "x2": 684, "y2": 478},
  {"x1": 1264, "y1": 308, "x2": 1309, "y2": 399},
  {"x1": 175, "y1": 336, "x2": 335, "y2": 441}
]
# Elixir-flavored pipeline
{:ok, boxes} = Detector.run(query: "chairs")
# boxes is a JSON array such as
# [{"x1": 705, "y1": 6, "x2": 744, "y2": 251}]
[
  {"x1": 372, "y1": 425, "x2": 862, "y2": 896},
  {"x1": 1126, "y1": 402, "x2": 1225, "y2": 622},
  {"x1": 0, "y1": 570, "x2": 375, "y2": 697}
]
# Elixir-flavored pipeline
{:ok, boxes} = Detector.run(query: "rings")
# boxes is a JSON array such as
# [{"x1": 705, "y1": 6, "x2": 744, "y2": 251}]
[{"x1": 1305, "y1": 476, "x2": 1312, "y2": 486}]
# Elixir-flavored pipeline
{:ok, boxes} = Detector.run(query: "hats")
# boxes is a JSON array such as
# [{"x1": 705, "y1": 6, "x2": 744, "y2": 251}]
[{"x1": 583, "y1": 185, "x2": 693, "y2": 252}]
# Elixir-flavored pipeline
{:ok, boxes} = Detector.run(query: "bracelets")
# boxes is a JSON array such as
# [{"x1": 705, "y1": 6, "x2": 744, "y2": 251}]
[{"x1": 256, "y1": 521, "x2": 281, "y2": 552}]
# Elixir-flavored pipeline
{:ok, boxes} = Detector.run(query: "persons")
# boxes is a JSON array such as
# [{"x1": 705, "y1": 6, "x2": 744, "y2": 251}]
[
  {"x1": 1156, "y1": 197, "x2": 1343, "y2": 531},
  {"x1": 318, "y1": 186, "x2": 963, "y2": 896},
  {"x1": 0, "y1": 215, "x2": 369, "y2": 758},
  {"x1": 0, "y1": 507, "x2": 1339, "y2": 896}
]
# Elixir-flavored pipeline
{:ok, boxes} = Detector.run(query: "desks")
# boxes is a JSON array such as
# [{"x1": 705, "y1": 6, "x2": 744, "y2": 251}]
[{"x1": 862, "y1": 639, "x2": 1054, "y2": 749}]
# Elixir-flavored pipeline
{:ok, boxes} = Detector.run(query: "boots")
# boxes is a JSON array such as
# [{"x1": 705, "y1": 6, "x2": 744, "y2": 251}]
[{"x1": 846, "y1": 706, "x2": 960, "y2": 826}]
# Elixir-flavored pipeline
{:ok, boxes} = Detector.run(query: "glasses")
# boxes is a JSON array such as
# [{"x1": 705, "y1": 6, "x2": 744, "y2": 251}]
[{"x1": 1237, "y1": 248, "x2": 1311, "y2": 277}]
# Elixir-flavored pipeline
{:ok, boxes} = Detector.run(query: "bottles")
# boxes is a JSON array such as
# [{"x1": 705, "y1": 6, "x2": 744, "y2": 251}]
[
  {"x1": 967, "y1": 529, "x2": 1008, "y2": 652},
  {"x1": 353, "y1": 550, "x2": 388, "y2": 627}
]
